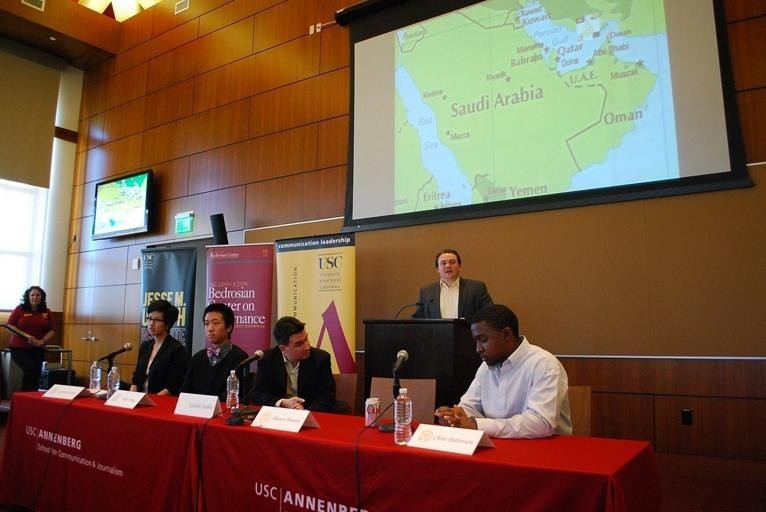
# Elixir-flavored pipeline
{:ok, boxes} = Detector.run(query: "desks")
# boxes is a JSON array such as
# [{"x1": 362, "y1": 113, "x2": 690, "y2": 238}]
[{"x1": 8, "y1": 386, "x2": 654, "y2": 512}]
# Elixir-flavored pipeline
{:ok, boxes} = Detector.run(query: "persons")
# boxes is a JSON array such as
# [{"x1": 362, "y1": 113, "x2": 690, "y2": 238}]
[
  {"x1": 253, "y1": 316, "x2": 336, "y2": 412},
  {"x1": 128, "y1": 300, "x2": 187, "y2": 396},
  {"x1": 5, "y1": 286, "x2": 56, "y2": 391},
  {"x1": 409, "y1": 248, "x2": 494, "y2": 321},
  {"x1": 192, "y1": 303, "x2": 249, "y2": 402},
  {"x1": 433, "y1": 303, "x2": 573, "y2": 439}
]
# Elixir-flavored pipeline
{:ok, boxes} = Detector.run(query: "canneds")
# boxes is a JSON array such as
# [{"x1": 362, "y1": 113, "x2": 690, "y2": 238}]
[{"x1": 364, "y1": 397, "x2": 381, "y2": 428}]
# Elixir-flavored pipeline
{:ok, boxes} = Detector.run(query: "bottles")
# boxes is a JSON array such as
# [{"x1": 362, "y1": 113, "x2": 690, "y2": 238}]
[
  {"x1": 394, "y1": 388, "x2": 412, "y2": 445},
  {"x1": 41, "y1": 360, "x2": 48, "y2": 387},
  {"x1": 88, "y1": 361, "x2": 101, "y2": 390},
  {"x1": 106, "y1": 366, "x2": 120, "y2": 399},
  {"x1": 226, "y1": 369, "x2": 240, "y2": 409}
]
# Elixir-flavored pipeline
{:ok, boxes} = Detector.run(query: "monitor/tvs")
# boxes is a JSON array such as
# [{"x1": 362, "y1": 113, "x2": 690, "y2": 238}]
[{"x1": 90, "y1": 170, "x2": 152, "y2": 241}]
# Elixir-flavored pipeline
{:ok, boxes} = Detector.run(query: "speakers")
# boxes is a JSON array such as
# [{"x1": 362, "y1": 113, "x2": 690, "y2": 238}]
[{"x1": 37, "y1": 368, "x2": 75, "y2": 390}]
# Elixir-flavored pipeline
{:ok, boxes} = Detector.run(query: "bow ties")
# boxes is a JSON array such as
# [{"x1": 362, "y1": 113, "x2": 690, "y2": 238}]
[{"x1": 206, "y1": 346, "x2": 221, "y2": 358}]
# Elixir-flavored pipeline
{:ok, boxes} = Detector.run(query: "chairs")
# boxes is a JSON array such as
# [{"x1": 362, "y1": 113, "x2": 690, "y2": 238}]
[
  {"x1": 332, "y1": 373, "x2": 358, "y2": 416},
  {"x1": 368, "y1": 376, "x2": 436, "y2": 425},
  {"x1": 567, "y1": 384, "x2": 592, "y2": 437}
]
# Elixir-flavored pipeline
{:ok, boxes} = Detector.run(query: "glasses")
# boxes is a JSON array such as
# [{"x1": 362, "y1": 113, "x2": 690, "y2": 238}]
[{"x1": 144, "y1": 317, "x2": 163, "y2": 322}]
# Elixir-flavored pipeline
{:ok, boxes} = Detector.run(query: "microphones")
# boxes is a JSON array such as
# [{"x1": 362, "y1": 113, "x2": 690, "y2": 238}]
[
  {"x1": 97, "y1": 342, "x2": 131, "y2": 362},
  {"x1": 391, "y1": 350, "x2": 408, "y2": 373},
  {"x1": 235, "y1": 350, "x2": 263, "y2": 370},
  {"x1": 392, "y1": 302, "x2": 424, "y2": 320}
]
